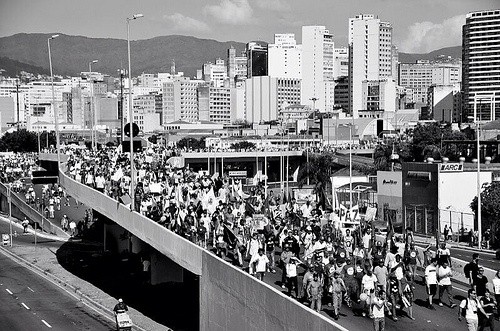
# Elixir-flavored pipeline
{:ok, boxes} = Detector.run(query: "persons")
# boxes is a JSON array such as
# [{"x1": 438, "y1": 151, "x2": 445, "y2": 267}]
[{"x1": 0, "y1": 142, "x2": 500, "y2": 331}]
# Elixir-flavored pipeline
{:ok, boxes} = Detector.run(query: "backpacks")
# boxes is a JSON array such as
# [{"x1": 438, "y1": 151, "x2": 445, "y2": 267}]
[
  {"x1": 459, "y1": 298, "x2": 477, "y2": 316},
  {"x1": 464, "y1": 264, "x2": 471, "y2": 278}
]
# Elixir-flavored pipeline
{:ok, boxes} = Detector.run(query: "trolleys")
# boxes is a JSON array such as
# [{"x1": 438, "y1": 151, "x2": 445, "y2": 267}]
[{"x1": 115, "y1": 309, "x2": 133, "y2": 331}]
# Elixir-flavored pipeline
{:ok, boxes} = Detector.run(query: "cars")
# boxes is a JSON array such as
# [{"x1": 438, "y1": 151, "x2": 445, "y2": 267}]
[{"x1": 447, "y1": 227, "x2": 479, "y2": 247}]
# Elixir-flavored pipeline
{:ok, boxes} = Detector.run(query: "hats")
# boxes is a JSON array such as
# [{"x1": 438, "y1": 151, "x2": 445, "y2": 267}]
[
  {"x1": 378, "y1": 258, "x2": 385, "y2": 263},
  {"x1": 334, "y1": 271, "x2": 341, "y2": 274},
  {"x1": 377, "y1": 240, "x2": 383, "y2": 247}
]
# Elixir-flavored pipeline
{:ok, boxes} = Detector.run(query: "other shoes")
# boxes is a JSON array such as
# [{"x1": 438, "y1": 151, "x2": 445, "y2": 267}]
[
  {"x1": 450, "y1": 303, "x2": 457, "y2": 309},
  {"x1": 439, "y1": 302, "x2": 443, "y2": 307},
  {"x1": 334, "y1": 315, "x2": 339, "y2": 320},
  {"x1": 267, "y1": 269, "x2": 270, "y2": 272},
  {"x1": 272, "y1": 270, "x2": 276, "y2": 273},
  {"x1": 393, "y1": 315, "x2": 398, "y2": 320},
  {"x1": 429, "y1": 304, "x2": 434, "y2": 310}
]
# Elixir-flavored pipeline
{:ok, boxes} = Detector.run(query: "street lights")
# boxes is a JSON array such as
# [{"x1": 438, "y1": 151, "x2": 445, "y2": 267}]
[
  {"x1": 88, "y1": 60, "x2": 99, "y2": 72},
  {"x1": 47, "y1": 34, "x2": 61, "y2": 170},
  {"x1": 0, "y1": 181, "x2": 24, "y2": 247},
  {"x1": 343, "y1": 123, "x2": 352, "y2": 209},
  {"x1": 284, "y1": 128, "x2": 289, "y2": 202},
  {"x1": 125, "y1": 12, "x2": 145, "y2": 211},
  {"x1": 468, "y1": 115, "x2": 482, "y2": 248}
]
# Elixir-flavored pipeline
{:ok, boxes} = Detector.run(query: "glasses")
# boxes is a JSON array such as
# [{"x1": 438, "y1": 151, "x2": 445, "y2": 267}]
[
  {"x1": 471, "y1": 293, "x2": 476, "y2": 295},
  {"x1": 479, "y1": 270, "x2": 484, "y2": 272}
]
